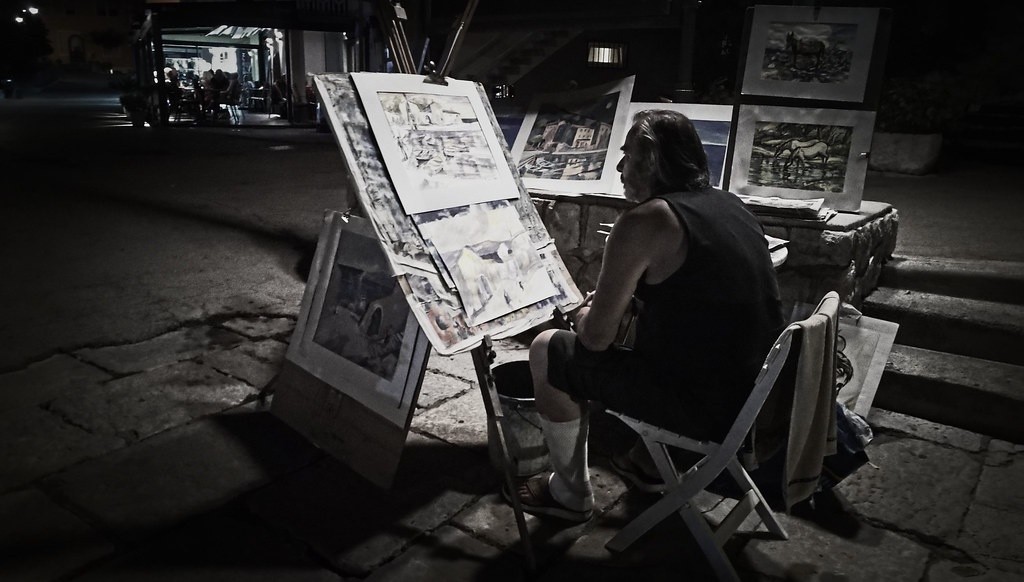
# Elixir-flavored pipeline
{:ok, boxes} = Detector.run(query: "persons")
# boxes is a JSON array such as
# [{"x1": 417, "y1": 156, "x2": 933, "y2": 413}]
[
  {"x1": 504, "y1": 110, "x2": 792, "y2": 522},
  {"x1": 118, "y1": 60, "x2": 242, "y2": 128}
]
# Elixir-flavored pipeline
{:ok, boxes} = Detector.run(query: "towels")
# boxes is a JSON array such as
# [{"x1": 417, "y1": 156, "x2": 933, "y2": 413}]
[{"x1": 751, "y1": 291, "x2": 844, "y2": 505}]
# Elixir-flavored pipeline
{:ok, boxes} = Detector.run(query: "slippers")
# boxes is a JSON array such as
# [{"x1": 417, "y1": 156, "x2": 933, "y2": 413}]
[
  {"x1": 501, "y1": 471, "x2": 593, "y2": 521},
  {"x1": 610, "y1": 448, "x2": 666, "y2": 493}
]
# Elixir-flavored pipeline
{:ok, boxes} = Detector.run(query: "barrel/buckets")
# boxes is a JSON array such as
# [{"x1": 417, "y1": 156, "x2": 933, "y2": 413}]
[{"x1": 488, "y1": 361, "x2": 552, "y2": 476}]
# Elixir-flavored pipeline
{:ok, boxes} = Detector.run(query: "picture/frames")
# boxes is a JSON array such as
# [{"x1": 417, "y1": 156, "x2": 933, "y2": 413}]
[
  {"x1": 728, "y1": 102, "x2": 878, "y2": 214},
  {"x1": 509, "y1": 69, "x2": 637, "y2": 189},
  {"x1": 351, "y1": 68, "x2": 526, "y2": 207},
  {"x1": 787, "y1": 300, "x2": 902, "y2": 422},
  {"x1": 284, "y1": 208, "x2": 432, "y2": 436},
  {"x1": 619, "y1": 105, "x2": 730, "y2": 194},
  {"x1": 739, "y1": 2, "x2": 882, "y2": 107}
]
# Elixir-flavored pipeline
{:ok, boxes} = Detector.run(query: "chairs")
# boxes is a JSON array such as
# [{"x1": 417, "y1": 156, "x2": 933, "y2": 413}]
[{"x1": 600, "y1": 289, "x2": 842, "y2": 582}]
